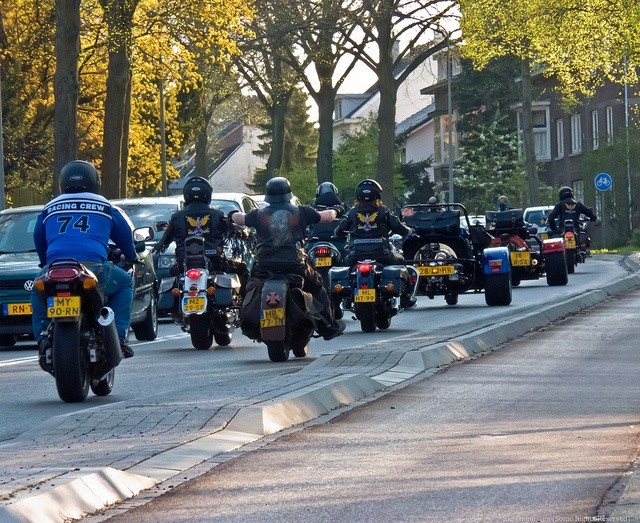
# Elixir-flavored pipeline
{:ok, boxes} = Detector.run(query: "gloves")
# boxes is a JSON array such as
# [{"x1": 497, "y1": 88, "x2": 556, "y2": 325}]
[{"x1": 327, "y1": 204, "x2": 344, "y2": 217}]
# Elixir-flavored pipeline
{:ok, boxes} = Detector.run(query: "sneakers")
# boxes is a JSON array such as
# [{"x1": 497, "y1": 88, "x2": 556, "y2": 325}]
[
  {"x1": 403, "y1": 297, "x2": 417, "y2": 308},
  {"x1": 120, "y1": 340, "x2": 134, "y2": 358},
  {"x1": 38, "y1": 338, "x2": 53, "y2": 371}
]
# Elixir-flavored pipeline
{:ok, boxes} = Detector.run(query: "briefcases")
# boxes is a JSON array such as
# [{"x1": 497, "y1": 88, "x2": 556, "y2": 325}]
[
  {"x1": 307, "y1": 220, "x2": 351, "y2": 241},
  {"x1": 403, "y1": 210, "x2": 461, "y2": 235},
  {"x1": 485, "y1": 209, "x2": 525, "y2": 228}
]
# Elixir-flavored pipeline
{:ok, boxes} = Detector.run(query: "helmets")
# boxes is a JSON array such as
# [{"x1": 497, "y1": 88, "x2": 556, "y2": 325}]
[
  {"x1": 559, "y1": 186, "x2": 573, "y2": 200},
  {"x1": 59, "y1": 159, "x2": 100, "y2": 192},
  {"x1": 264, "y1": 176, "x2": 292, "y2": 204},
  {"x1": 183, "y1": 176, "x2": 213, "y2": 205},
  {"x1": 316, "y1": 181, "x2": 338, "y2": 197},
  {"x1": 355, "y1": 178, "x2": 382, "y2": 201}
]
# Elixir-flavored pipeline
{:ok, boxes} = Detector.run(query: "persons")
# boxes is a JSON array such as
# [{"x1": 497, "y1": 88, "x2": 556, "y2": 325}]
[
  {"x1": 223, "y1": 176, "x2": 346, "y2": 340},
  {"x1": 541, "y1": 217, "x2": 547, "y2": 224},
  {"x1": 150, "y1": 176, "x2": 250, "y2": 324},
  {"x1": 427, "y1": 196, "x2": 446, "y2": 211},
  {"x1": 546, "y1": 185, "x2": 597, "y2": 256},
  {"x1": 159, "y1": 222, "x2": 168, "y2": 231},
  {"x1": 487, "y1": 222, "x2": 496, "y2": 230},
  {"x1": 334, "y1": 178, "x2": 415, "y2": 309},
  {"x1": 302, "y1": 181, "x2": 352, "y2": 258},
  {"x1": 486, "y1": 194, "x2": 539, "y2": 273},
  {"x1": 30, "y1": 158, "x2": 140, "y2": 372}
]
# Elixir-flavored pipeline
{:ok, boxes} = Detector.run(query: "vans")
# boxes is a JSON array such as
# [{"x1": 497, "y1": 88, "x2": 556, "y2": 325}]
[{"x1": 524, "y1": 206, "x2": 559, "y2": 240}]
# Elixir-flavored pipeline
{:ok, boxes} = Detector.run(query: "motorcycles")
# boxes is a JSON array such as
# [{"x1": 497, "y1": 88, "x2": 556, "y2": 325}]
[
  {"x1": 154, "y1": 229, "x2": 245, "y2": 350},
  {"x1": 231, "y1": 203, "x2": 342, "y2": 362},
  {"x1": 333, "y1": 228, "x2": 419, "y2": 332},
  {"x1": 304, "y1": 241, "x2": 345, "y2": 318},
  {"x1": 34, "y1": 245, "x2": 144, "y2": 401},
  {"x1": 553, "y1": 216, "x2": 594, "y2": 273}
]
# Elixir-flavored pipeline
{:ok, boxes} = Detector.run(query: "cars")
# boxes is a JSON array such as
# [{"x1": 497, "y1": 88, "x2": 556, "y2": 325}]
[
  {"x1": 110, "y1": 197, "x2": 184, "y2": 322},
  {"x1": 250, "y1": 194, "x2": 304, "y2": 211},
  {"x1": 0, "y1": 205, "x2": 158, "y2": 348},
  {"x1": 171, "y1": 192, "x2": 261, "y2": 271}
]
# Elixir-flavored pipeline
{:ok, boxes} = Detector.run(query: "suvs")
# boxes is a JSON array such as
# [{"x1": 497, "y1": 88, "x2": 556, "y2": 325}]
[
  {"x1": 479, "y1": 210, "x2": 567, "y2": 286},
  {"x1": 403, "y1": 204, "x2": 512, "y2": 306}
]
